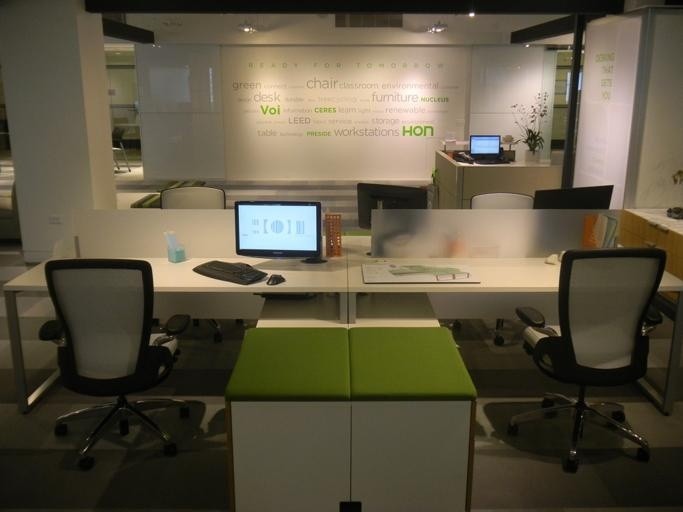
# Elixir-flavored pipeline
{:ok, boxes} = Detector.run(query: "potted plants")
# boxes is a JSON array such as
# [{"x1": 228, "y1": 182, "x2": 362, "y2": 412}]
[{"x1": 512, "y1": 91, "x2": 551, "y2": 166}]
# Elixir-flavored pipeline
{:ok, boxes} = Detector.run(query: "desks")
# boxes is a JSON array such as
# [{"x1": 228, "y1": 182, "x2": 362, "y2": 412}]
[
  {"x1": 435, "y1": 149, "x2": 564, "y2": 209},
  {"x1": 349, "y1": 234, "x2": 683, "y2": 417},
  {"x1": 225, "y1": 293, "x2": 477, "y2": 511},
  {"x1": 619, "y1": 206, "x2": 683, "y2": 303},
  {"x1": 3, "y1": 235, "x2": 347, "y2": 415}
]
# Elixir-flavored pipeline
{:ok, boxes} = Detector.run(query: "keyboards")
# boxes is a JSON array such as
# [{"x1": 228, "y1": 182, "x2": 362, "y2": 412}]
[{"x1": 193, "y1": 260, "x2": 267, "y2": 285}]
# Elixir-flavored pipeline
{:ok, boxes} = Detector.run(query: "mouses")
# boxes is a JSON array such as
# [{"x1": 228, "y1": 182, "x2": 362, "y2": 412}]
[{"x1": 267, "y1": 274, "x2": 286, "y2": 286}]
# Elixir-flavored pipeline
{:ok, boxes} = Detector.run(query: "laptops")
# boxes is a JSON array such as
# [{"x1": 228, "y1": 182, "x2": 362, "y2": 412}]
[{"x1": 469, "y1": 134, "x2": 510, "y2": 165}]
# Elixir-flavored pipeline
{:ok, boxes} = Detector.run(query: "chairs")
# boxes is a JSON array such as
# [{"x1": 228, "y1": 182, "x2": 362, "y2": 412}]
[
  {"x1": 43, "y1": 259, "x2": 190, "y2": 472},
  {"x1": 470, "y1": 192, "x2": 534, "y2": 209},
  {"x1": 112, "y1": 126, "x2": 133, "y2": 173},
  {"x1": 159, "y1": 186, "x2": 226, "y2": 209},
  {"x1": 506, "y1": 248, "x2": 668, "y2": 463}
]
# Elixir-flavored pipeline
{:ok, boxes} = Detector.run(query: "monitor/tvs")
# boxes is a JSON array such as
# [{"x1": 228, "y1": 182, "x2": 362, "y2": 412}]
[
  {"x1": 234, "y1": 201, "x2": 322, "y2": 258},
  {"x1": 535, "y1": 185, "x2": 615, "y2": 209},
  {"x1": 356, "y1": 182, "x2": 430, "y2": 230}
]
